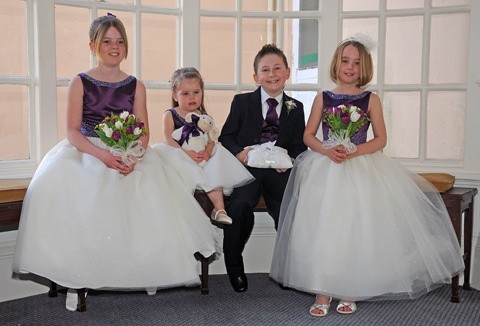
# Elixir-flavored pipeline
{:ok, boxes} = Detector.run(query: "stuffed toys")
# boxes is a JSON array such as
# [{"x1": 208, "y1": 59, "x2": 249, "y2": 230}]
[{"x1": 171, "y1": 111, "x2": 215, "y2": 165}]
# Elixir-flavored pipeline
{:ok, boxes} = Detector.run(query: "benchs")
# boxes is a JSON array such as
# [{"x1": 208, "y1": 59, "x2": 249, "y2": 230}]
[{"x1": 0, "y1": 174, "x2": 477, "y2": 312}]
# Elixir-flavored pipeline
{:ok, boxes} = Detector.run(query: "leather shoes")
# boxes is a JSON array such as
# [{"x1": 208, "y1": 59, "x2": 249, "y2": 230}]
[{"x1": 229, "y1": 272, "x2": 248, "y2": 293}]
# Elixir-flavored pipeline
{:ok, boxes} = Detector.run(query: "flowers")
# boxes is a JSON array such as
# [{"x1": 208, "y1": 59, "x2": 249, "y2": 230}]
[
  {"x1": 284, "y1": 99, "x2": 296, "y2": 115},
  {"x1": 94, "y1": 111, "x2": 148, "y2": 167},
  {"x1": 322, "y1": 104, "x2": 372, "y2": 154}
]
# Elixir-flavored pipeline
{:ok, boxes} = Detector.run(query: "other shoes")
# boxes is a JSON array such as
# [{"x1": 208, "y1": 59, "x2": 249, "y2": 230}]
[{"x1": 211, "y1": 209, "x2": 233, "y2": 225}]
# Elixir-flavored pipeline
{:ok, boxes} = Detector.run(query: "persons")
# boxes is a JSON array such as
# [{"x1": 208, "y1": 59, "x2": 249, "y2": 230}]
[
  {"x1": 10, "y1": 12, "x2": 223, "y2": 312},
  {"x1": 162, "y1": 66, "x2": 256, "y2": 226},
  {"x1": 270, "y1": 37, "x2": 466, "y2": 317},
  {"x1": 219, "y1": 44, "x2": 307, "y2": 293}
]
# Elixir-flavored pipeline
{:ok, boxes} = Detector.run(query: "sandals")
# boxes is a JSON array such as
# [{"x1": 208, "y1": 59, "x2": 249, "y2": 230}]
[
  {"x1": 309, "y1": 293, "x2": 332, "y2": 316},
  {"x1": 336, "y1": 302, "x2": 357, "y2": 313}
]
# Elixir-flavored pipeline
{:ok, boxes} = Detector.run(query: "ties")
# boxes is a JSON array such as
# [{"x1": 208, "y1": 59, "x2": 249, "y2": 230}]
[{"x1": 265, "y1": 98, "x2": 279, "y2": 142}]
